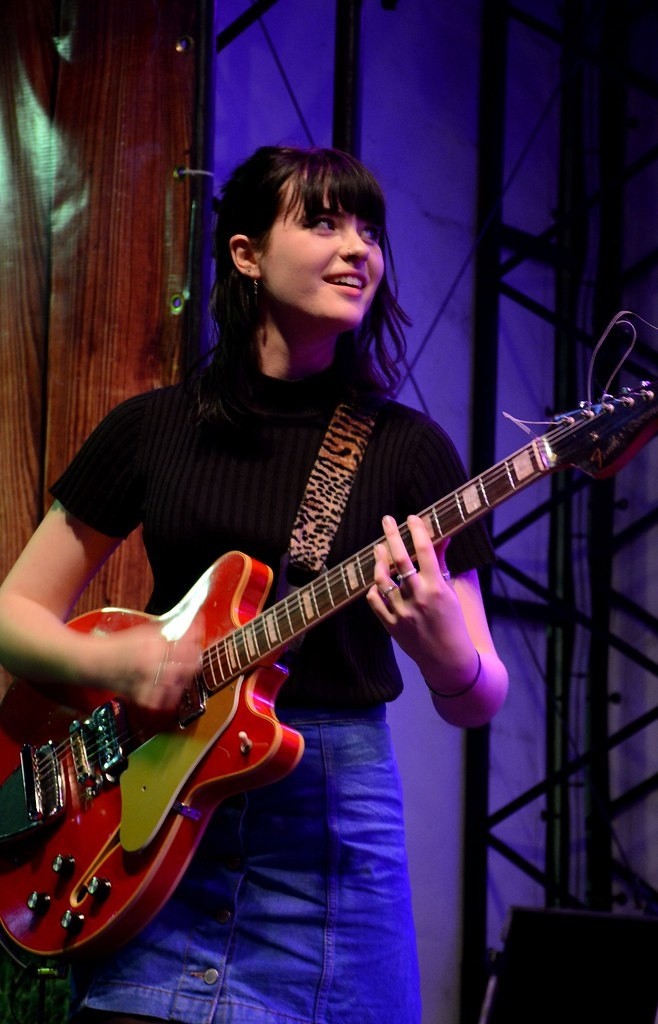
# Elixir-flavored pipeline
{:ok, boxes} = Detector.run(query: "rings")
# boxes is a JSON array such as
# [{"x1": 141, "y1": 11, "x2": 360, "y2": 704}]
[
  {"x1": 396, "y1": 568, "x2": 417, "y2": 579},
  {"x1": 382, "y1": 585, "x2": 398, "y2": 597},
  {"x1": 442, "y1": 570, "x2": 450, "y2": 580}
]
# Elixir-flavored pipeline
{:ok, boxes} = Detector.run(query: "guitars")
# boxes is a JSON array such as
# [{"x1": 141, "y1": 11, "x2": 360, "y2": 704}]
[{"x1": 0, "y1": 378, "x2": 658, "y2": 979}]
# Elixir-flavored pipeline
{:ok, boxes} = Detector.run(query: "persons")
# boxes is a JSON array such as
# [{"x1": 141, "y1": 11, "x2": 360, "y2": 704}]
[{"x1": 0, "y1": 148, "x2": 511, "y2": 1024}]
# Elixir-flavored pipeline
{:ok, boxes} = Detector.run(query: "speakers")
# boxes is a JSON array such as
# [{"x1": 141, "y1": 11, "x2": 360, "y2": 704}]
[{"x1": 483, "y1": 905, "x2": 658, "y2": 1024}]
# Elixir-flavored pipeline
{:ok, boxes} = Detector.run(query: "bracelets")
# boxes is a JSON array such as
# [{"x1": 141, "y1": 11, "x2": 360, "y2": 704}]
[{"x1": 424, "y1": 648, "x2": 482, "y2": 698}]
